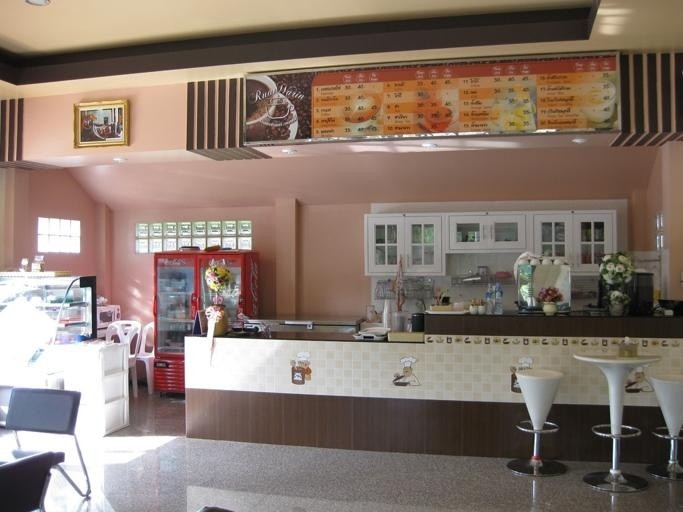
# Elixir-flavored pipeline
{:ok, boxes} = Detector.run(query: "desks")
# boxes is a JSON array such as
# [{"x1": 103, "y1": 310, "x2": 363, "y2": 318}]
[{"x1": 572, "y1": 353, "x2": 663, "y2": 495}]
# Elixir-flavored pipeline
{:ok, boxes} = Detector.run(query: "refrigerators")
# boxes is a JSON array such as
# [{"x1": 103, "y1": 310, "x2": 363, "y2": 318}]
[{"x1": 153, "y1": 249, "x2": 260, "y2": 394}]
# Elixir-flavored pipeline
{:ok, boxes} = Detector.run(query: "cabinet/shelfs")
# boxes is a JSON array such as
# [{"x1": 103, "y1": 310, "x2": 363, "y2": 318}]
[
  {"x1": 365, "y1": 214, "x2": 441, "y2": 276},
  {"x1": 533, "y1": 210, "x2": 617, "y2": 277},
  {"x1": 450, "y1": 211, "x2": 526, "y2": 253}
]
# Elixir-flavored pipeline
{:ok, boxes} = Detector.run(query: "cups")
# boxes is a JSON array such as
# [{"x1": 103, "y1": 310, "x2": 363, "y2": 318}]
[
  {"x1": 516, "y1": 258, "x2": 567, "y2": 266},
  {"x1": 575, "y1": 80, "x2": 616, "y2": 123},
  {"x1": 337, "y1": 89, "x2": 383, "y2": 132}
]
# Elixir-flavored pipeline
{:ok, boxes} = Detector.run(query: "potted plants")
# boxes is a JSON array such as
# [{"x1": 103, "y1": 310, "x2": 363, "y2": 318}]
[
  {"x1": 479, "y1": 298, "x2": 486, "y2": 315},
  {"x1": 469, "y1": 299, "x2": 478, "y2": 315}
]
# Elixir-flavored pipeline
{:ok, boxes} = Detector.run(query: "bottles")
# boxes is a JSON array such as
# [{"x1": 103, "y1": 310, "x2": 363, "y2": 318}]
[
  {"x1": 411, "y1": 313, "x2": 424, "y2": 333},
  {"x1": 495, "y1": 281, "x2": 503, "y2": 315},
  {"x1": 486, "y1": 282, "x2": 495, "y2": 315}
]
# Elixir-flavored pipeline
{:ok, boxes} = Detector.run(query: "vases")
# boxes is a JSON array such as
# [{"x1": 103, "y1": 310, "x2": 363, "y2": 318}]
[
  {"x1": 209, "y1": 293, "x2": 229, "y2": 337},
  {"x1": 541, "y1": 302, "x2": 557, "y2": 315},
  {"x1": 604, "y1": 287, "x2": 631, "y2": 316}
]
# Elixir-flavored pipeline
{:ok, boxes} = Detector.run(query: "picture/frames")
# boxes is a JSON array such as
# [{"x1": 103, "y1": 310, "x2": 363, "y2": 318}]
[{"x1": 73, "y1": 100, "x2": 130, "y2": 148}]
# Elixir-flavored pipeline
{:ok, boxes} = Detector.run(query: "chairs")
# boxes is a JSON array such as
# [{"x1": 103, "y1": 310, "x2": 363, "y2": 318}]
[
  {"x1": 0, "y1": 270, "x2": 153, "y2": 511},
  {"x1": 106, "y1": 320, "x2": 139, "y2": 398},
  {"x1": 140, "y1": 323, "x2": 156, "y2": 399}
]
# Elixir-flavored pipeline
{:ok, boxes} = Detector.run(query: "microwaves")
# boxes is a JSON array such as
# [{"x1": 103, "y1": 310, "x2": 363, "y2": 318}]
[{"x1": 97, "y1": 305, "x2": 121, "y2": 329}]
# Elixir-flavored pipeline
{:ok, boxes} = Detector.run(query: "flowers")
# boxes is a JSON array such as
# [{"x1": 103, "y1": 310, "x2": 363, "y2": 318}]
[
  {"x1": 536, "y1": 285, "x2": 564, "y2": 302},
  {"x1": 596, "y1": 250, "x2": 634, "y2": 288},
  {"x1": 204, "y1": 263, "x2": 236, "y2": 295}
]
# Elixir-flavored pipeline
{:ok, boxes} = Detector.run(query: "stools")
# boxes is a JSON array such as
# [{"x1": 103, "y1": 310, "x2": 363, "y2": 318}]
[
  {"x1": 645, "y1": 377, "x2": 683, "y2": 482},
  {"x1": 505, "y1": 368, "x2": 568, "y2": 477}
]
